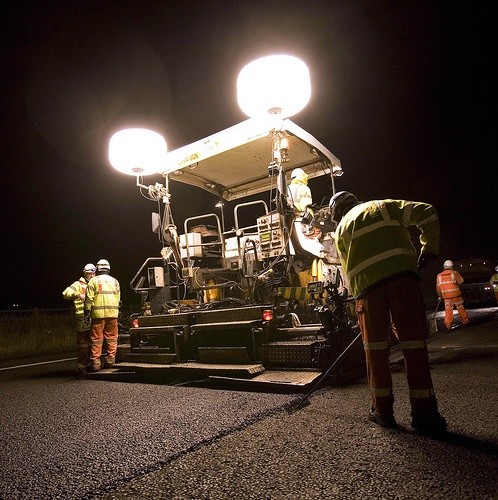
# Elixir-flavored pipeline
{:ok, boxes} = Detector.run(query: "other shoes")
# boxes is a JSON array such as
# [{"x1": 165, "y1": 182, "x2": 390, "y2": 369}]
[
  {"x1": 104, "y1": 363, "x2": 115, "y2": 368},
  {"x1": 409, "y1": 412, "x2": 448, "y2": 430},
  {"x1": 369, "y1": 410, "x2": 396, "y2": 427},
  {"x1": 445, "y1": 326, "x2": 451, "y2": 332},
  {"x1": 79, "y1": 369, "x2": 88, "y2": 377},
  {"x1": 94, "y1": 367, "x2": 100, "y2": 372},
  {"x1": 464, "y1": 322, "x2": 472, "y2": 328}
]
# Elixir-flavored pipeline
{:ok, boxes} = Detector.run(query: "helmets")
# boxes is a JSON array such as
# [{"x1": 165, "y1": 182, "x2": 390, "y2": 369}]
[
  {"x1": 96, "y1": 258, "x2": 110, "y2": 269},
  {"x1": 495, "y1": 265, "x2": 498, "y2": 271},
  {"x1": 83, "y1": 263, "x2": 96, "y2": 274},
  {"x1": 443, "y1": 260, "x2": 453, "y2": 267},
  {"x1": 329, "y1": 191, "x2": 356, "y2": 220}
]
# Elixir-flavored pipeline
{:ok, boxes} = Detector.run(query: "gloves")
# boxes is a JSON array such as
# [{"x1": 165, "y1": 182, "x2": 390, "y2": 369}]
[{"x1": 417, "y1": 254, "x2": 433, "y2": 271}]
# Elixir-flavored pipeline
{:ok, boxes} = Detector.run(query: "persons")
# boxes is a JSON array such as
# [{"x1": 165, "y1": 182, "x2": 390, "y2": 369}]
[
  {"x1": 85, "y1": 259, "x2": 122, "y2": 372},
  {"x1": 490, "y1": 267, "x2": 498, "y2": 302},
  {"x1": 436, "y1": 260, "x2": 470, "y2": 331},
  {"x1": 287, "y1": 167, "x2": 312, "y2": 213},
  {"x1": 62, "y1": 263, "x2": 97, "y2": 375},
  {"x1": 328, "y1": 190, "x2": 449, "y2": 430}
]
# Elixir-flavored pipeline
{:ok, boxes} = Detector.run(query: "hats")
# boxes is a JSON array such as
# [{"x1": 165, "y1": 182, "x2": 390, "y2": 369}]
[{"x1": 291, "y1": 168, "x2": 304, "y2": 178}]
